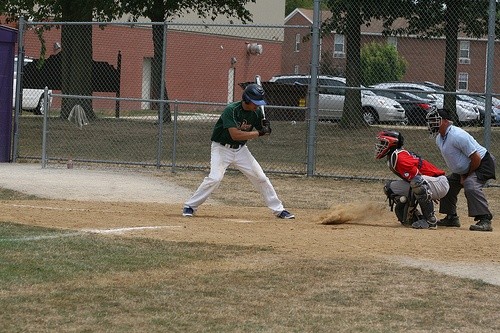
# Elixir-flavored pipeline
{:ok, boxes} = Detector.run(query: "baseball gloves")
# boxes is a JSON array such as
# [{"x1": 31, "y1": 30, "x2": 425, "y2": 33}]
[{"x1": 395, "y1": 200, "x2": 416, "y2": 227}]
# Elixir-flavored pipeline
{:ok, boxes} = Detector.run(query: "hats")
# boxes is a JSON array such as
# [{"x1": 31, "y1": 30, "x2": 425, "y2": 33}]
[{"x1": 438, "y1": 110, "x2": 454, "y2": 121}]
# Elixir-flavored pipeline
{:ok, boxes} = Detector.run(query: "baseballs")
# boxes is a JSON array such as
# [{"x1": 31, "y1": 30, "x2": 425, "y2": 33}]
[{"x1": 400, "y1": 196, "x2": 406, "y2": 202}]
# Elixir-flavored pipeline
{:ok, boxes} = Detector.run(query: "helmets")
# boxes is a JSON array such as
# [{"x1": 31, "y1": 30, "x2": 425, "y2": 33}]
[
  {"x1": 373, "y1": 130, "x2": 403, "y2": 160},
  {"x1": 244, "y1": 84, "x2": 266, "y2": 105}
]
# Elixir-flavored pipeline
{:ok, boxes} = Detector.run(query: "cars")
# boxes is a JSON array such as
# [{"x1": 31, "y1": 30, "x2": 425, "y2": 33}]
[
  {"x1": 369, "y1": 87, "x2": 437, "y2": 126},
  {"x1": 468, "y1": 93, "x2": 500, "y2": 121}
]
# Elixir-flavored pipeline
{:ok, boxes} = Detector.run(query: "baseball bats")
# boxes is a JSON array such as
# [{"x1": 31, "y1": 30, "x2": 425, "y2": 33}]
[{"x1": 255, "y1": 74, "x2": 270, "y2": 136}]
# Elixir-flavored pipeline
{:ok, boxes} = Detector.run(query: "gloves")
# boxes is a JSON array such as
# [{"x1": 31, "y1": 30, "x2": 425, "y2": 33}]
[
  {"x1": 262, "y1": 118, "x2": 270, "y2": 127},
  {"x1": 258, "y1": 127, "x2": 271, "y2": 135}
]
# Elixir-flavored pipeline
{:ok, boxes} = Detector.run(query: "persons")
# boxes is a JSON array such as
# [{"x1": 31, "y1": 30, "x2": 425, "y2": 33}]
[
  {"x1": 376, "y1": 129, "x2": 450, "y2": 230},
  {"x1": 183, "y1": 84, "x2": 296, "y2": 219},
  {"x1": 426, "y1": 105, "x2": 496, "y2": 231}
]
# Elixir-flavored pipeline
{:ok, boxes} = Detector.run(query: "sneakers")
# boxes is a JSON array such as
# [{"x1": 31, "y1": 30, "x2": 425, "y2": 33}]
[
  {"x1": 279, "y1": 210, "x2": 295, "y2": 218},
  {"x1": 437, "y1": 215, "x2": 461, "y2": 227},
  {"x1": 183, "y1": 207, "x2": 193, "y2": 216},
  {"x1": 411, "y1": 220, "x2": 437, "y2": 230},
  {"x1": 470, "y1": 218, "x2": 492, "y2": 231}
]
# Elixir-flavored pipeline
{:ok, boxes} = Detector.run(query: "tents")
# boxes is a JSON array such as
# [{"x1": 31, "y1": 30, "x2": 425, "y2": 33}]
[{"x1": 0, "y1": 25, "x2": 19, "y2": 162}]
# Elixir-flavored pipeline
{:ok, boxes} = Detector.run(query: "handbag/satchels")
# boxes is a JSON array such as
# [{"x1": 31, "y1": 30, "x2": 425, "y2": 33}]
[{"x1": 475, "y1": 153, "x2": 496, "y2": 181}]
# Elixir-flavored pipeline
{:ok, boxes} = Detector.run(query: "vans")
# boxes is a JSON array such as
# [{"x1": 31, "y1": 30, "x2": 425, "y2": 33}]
[
  {"x1": 12, "y1": 54, "x2": 53, "y2": 115},
  {"x1": 266, "y1": 73, "x2": 406, "y2": 125}
]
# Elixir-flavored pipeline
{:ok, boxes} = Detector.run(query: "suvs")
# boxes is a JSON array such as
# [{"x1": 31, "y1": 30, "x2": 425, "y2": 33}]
[{"x1": 372, "y1": 81, "x2": 496, "y2": 126}]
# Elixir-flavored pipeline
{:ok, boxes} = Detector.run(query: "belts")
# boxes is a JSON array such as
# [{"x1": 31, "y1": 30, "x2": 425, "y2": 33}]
[{"x1": 221, "y1": 142, "x2": 242, "y2": 149}]
